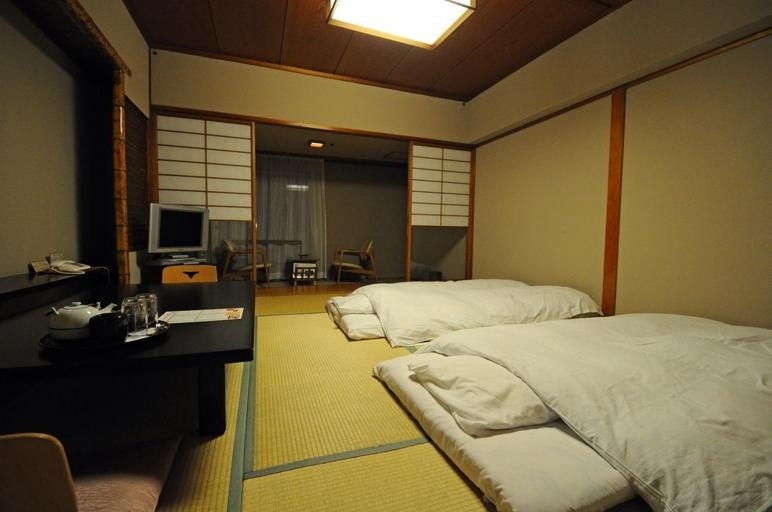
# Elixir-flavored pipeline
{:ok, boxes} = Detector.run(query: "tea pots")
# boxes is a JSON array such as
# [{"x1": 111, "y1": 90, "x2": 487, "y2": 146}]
[{"x1": 46, "y1": 302, "x2": 117, "y2": 340}]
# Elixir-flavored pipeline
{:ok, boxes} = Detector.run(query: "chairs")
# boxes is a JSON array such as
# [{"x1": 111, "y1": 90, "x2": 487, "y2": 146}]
[
  {"x1": 0, "y1": 432, "x2": 79, "y2": 512},
  {"x1": 221, "y1": 239, "x2": 272, "y2": 288},
  {"x1": 332, "y1": 240, "x2": 378, "y2": 285},
  {"x1": 161, "y1": 265, "x2": 218, "y2": 285}
]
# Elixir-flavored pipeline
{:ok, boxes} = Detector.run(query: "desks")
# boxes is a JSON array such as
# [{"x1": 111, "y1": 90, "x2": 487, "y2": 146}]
[
  {"x1": 0, "y1": 281, "x2": 257, "y2": 436},
  {"x1": 287, "y1": 257, "x2": 320, "y2": 287}
]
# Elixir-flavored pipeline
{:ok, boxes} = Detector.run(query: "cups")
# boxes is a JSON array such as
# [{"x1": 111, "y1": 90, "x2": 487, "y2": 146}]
[
  {"x1": 89, "y1": 313, "x2": 128, "y2": 345},
  {"x1": 120, "y1": 293, "x2": 158, "y2": 336}
]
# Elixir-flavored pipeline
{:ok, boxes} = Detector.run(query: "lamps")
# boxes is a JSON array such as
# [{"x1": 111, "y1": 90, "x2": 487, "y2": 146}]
[{"x1": 325, "y1": 0, "x2": 477, "y2": 50}]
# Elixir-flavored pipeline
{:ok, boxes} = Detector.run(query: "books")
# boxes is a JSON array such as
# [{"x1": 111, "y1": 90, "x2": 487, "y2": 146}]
[{"x1": 161, "y1": 252, "x2": 208, "y2": 265}]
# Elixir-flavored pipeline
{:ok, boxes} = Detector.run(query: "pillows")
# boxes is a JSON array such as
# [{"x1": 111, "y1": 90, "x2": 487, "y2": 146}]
[
  {"x1": 331, "y1": 294, "x2": 375, "y2": 315},
  {"x1": 409, "y1": 351, "x2": 559, "y2": 436}
]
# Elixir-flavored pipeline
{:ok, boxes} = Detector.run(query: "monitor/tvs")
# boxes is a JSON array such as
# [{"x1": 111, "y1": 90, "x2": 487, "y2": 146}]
[{"x1": 147, "y1": 203, "x2": 210, "y2": 265}]
